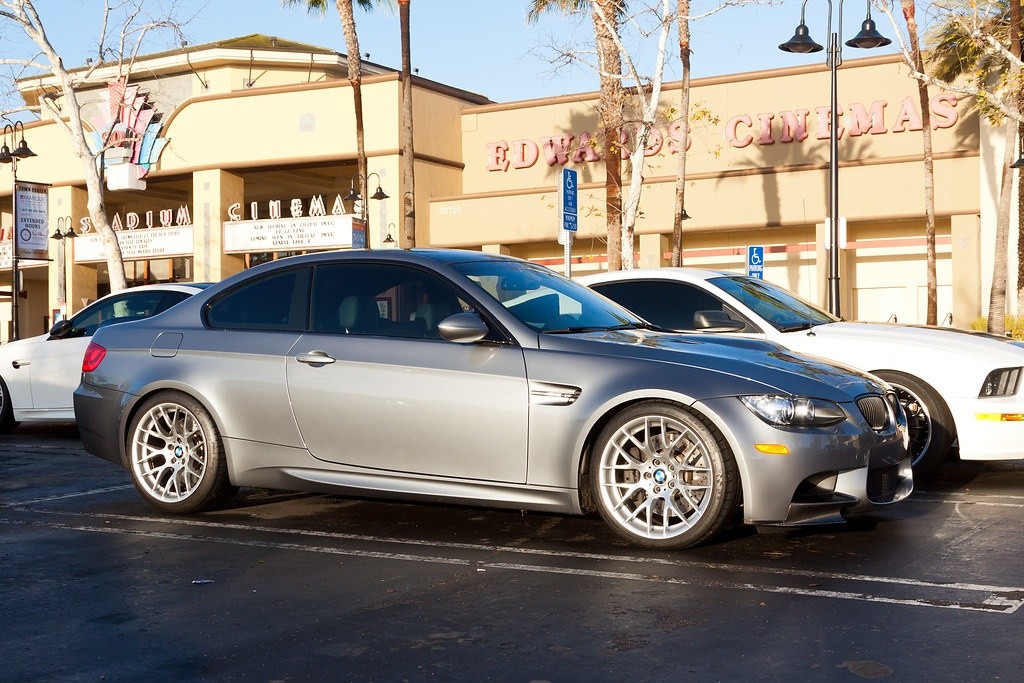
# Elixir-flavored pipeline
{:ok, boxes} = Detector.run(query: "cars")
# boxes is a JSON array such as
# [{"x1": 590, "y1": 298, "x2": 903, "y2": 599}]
[{"x1": 0, "y1": 248, "x2": 1024, "y2": 551}]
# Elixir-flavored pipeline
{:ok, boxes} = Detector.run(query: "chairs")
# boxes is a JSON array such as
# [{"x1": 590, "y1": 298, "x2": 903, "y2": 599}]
[
  {"x1": 341, "y1": 296, "x2": 407, "y2": 333},
  {"x1": 416, "y1": 302, "x2": 458, "y2": 341}
]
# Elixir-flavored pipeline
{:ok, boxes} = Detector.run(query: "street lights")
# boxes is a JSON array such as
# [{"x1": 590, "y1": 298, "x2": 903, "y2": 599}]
[
  {"x1": 777, "y1": 0, "x2": 892, "y2": 319},
  {"x1": 344, "y1": 172, "x2": 398, "y2": 248},
  {"x1": 0, "y1": 121, "x2": 37, "y2": 341}
]
